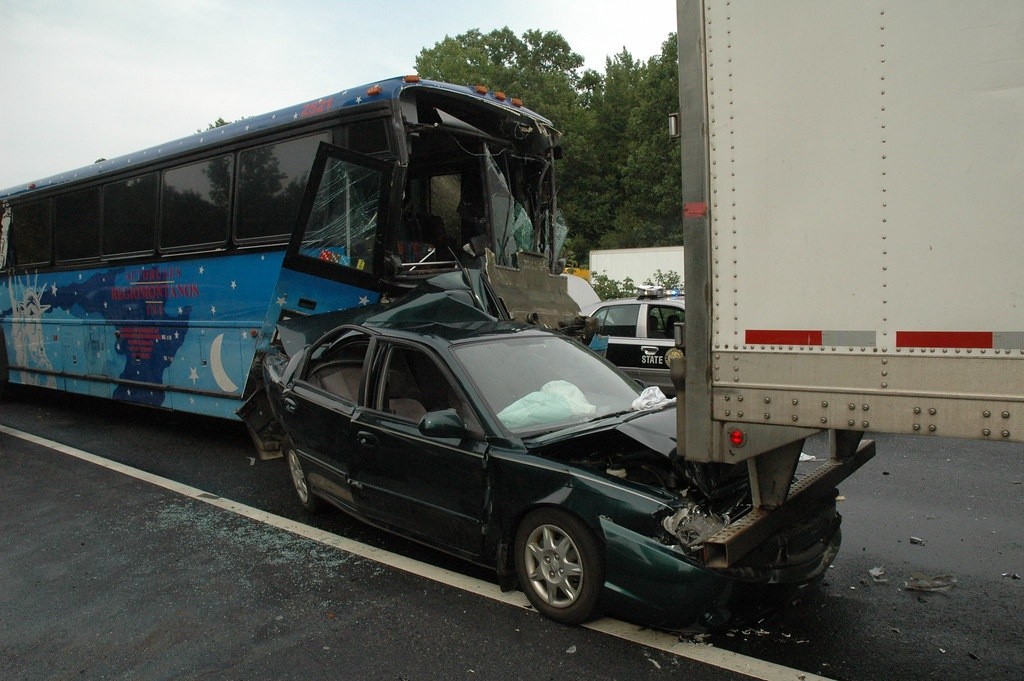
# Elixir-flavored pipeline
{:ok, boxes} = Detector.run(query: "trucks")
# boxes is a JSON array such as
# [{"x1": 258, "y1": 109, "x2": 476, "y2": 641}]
[{"x1": 675, "y1": 0, "x2": 1024, "y2": 464}]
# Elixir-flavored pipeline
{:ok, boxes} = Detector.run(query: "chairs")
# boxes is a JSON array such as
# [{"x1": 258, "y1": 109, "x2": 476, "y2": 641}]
[
  {"x1": 442, "y1": 379, "x2": 486, "y2": 442},
  {"x1": 411, "y1": 215, "x2": 452, "y2": 263},
  {"x1": 666, "y1": 314, "x2": 682, "y2": 339},
  {"x1": 383, "y1": 352, "x2": 427, "y2": 422},
  {"x1": 647, "y1": 315, "x2": 659, "y2": 338}
]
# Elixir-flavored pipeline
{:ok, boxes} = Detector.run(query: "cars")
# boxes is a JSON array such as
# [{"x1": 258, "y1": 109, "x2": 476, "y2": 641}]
[
  {"x1": 226, "y1": 281, "x2": 844, "y2": 634},
  {"x1": 571, "y1": 286, "x2": 686, "y2": 387}
]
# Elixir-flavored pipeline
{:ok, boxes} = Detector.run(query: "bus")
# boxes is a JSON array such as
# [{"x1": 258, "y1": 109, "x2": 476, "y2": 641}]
[{"x1": 0, "y1": 75, "x2": 553, "y2": 422}]
